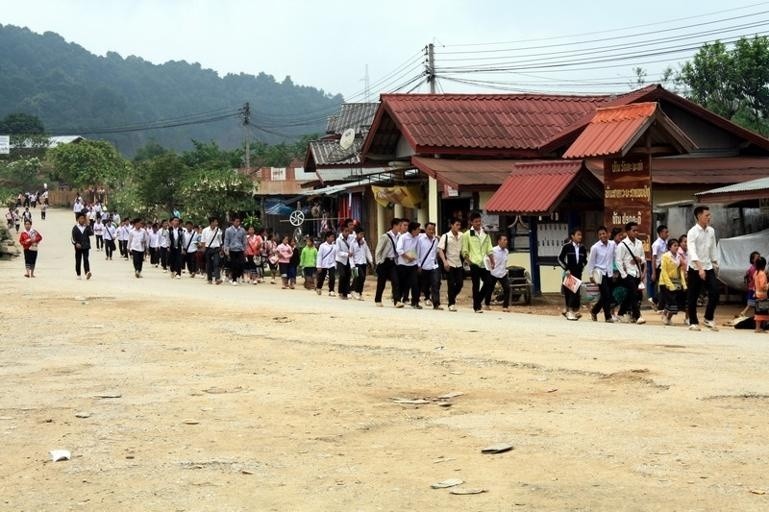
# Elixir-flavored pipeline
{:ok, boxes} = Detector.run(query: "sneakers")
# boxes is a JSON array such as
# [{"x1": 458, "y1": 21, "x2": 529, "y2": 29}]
[
  {"x1": 561, "y1": 308, "x2": 583, "y2": 321},
  {"x1": 605, "y1": 314, "x2": 647, "y2": 325},
  {"x1": 648, "y1": 297, "x2": 720, "y2": 333},
  {"x1": 474, "y1": 306, "x2": 512, "y2": 313},
  {"x1": 589, "y1": 309, "x2": 597, "y2": 322},
  {"x1": 76, "y1": 274, "x2": 82, "y2": 280},
  {"x1": 85, "y1": 272, "x2": 92, "y2": 280},
  {"x1": 374, "y1": 298, "x2": 457, "y2": 312},
  {"x1": 316, "y1": 287, "x2": 365, "y2": 302},
  {"x1": 96, "y1": 248, "x2": 276, "y2": 293}
]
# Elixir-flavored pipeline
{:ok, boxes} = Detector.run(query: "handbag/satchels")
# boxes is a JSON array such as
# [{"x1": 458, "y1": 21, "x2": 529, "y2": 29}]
[{"x1": 111, "y1": 240, "x2": 117, "y2": 250}]
